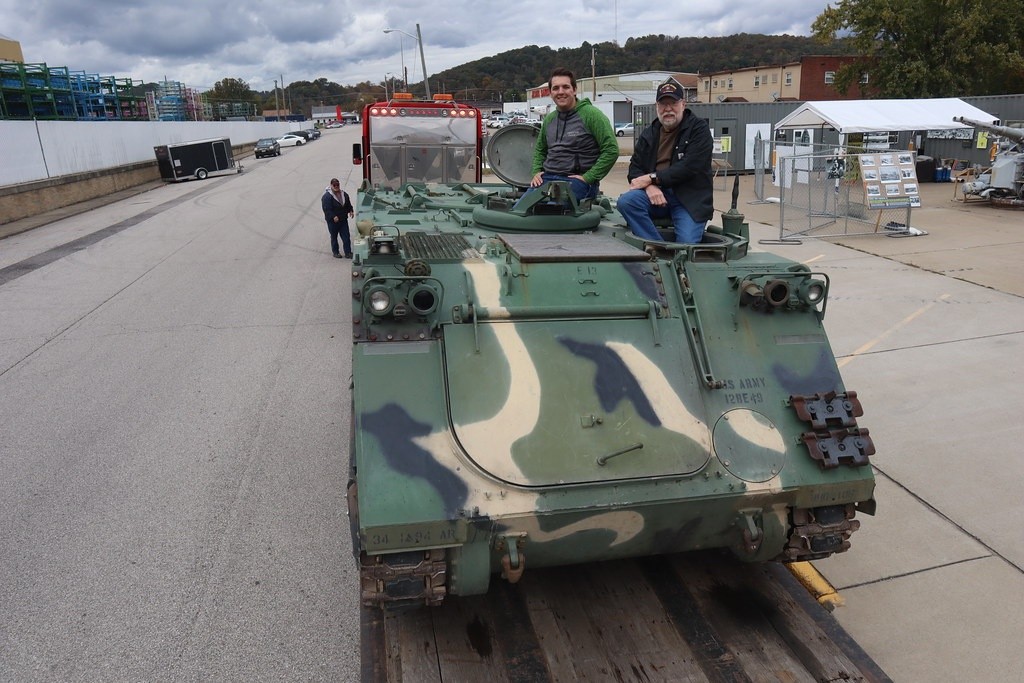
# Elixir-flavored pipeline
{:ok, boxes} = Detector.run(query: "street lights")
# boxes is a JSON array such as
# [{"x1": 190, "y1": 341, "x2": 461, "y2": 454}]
[
  {"x1": 370, "y1": 75, "x2": 388, "y2": 102},
  {"x1": 383, "y1": 23, "x2": 432, "y2": 101}
]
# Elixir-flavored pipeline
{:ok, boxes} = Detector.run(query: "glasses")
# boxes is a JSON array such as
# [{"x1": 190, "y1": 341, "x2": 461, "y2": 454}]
[
  {"x1": 332, "y1": 183, "x2": 340, "y2": 187},
  {"x1": 657, "y1": 97, "x2": 682, "y2": 107}
]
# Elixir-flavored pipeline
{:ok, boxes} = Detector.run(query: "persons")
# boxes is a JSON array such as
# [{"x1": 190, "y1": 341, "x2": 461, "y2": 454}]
[
  {"x1": 513, "y1": 67, "x2": 619, "y2": 210},
  {"x1": 321, "y1": 178, "x2": 354, "y2": 259},
  {"x1": 617, "y1": 83, "x2": 714, "y2": 243}
]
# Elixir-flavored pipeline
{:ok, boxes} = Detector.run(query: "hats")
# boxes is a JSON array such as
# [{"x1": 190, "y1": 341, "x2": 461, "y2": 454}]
[{"x1": 656, "y1": 82, "x2": 683, "y2": 101}]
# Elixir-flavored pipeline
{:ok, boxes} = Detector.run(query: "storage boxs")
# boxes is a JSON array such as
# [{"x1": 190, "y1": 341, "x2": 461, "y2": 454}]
[{"x1": 934, "y1": 167, "x2": 951, "y2": 182}]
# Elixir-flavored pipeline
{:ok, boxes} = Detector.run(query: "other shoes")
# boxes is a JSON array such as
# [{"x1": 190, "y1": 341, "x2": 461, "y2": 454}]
[
  {"x1": 333, "y1": 253, "x2": 342, "y2": 259},
  {"x1": 345, "y1": 252, "x2": 353, "y2": 258}
]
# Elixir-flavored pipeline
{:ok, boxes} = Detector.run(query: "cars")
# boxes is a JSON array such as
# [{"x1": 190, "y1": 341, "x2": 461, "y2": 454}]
[
  {"x1": 253, "y1": 138, "x2": 281, "y2": 159},
  {"x1": 325, "y1": 121, "x2": 345, "y2": 128},
  {"x1": 615, "y1": 123, "x2": 634, "y2": 137},
  {"x1": 481, "y1": 116, "x2": 542, "y2": 129},
  {"x1": 276, "y1": 129, "x2": 321, "y2": 148}
]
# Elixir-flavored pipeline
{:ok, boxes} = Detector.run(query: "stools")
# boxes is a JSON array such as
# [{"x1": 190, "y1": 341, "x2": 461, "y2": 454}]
[{"x1": 650, "y1": 217, "x2": 674, "y2": 226}]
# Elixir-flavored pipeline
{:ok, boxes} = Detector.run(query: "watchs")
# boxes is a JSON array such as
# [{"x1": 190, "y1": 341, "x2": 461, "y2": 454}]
[{"x1": 650, "y1": 172, "x2": 656, "y2": 184}]
